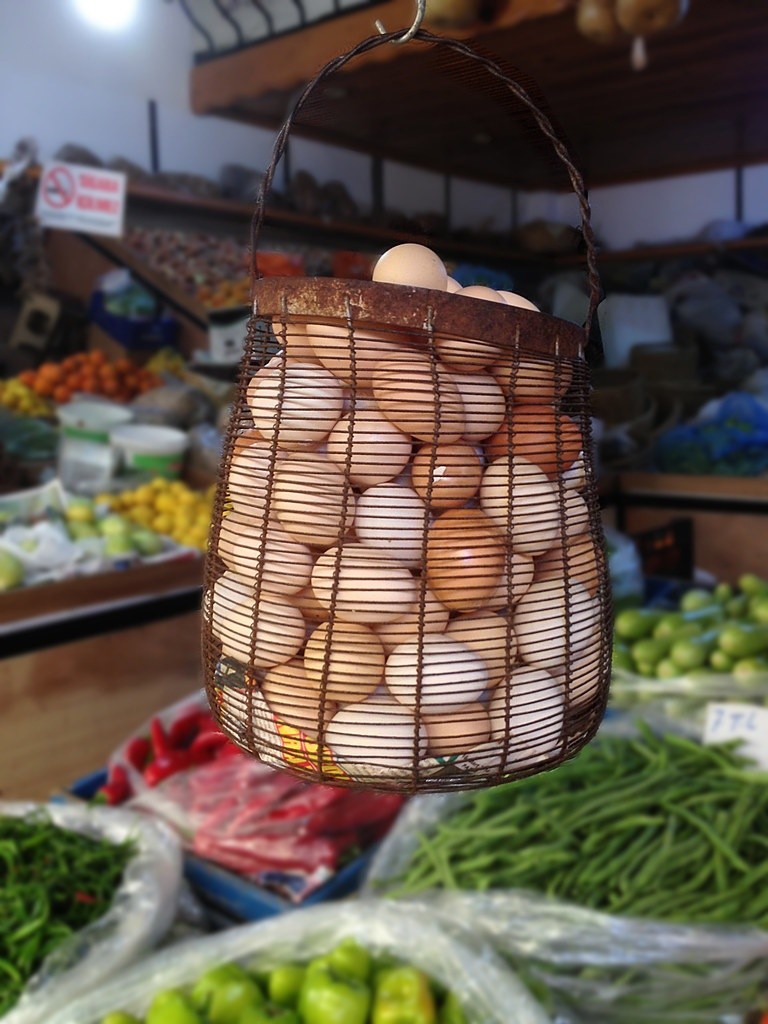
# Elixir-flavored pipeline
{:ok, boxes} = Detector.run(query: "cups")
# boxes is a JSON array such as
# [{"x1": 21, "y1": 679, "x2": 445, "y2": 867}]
[
  {"x1": 108, "y1": 423, "x2": 188, "y2": 482},
  {"x1": 58, "y1": 401, "x2": 132, "y2": 458}
]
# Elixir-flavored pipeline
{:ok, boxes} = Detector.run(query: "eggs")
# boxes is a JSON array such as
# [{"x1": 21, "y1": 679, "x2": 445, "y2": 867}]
[{"x1": 204, "y1": 238, "x2": 604, "y2": 781}]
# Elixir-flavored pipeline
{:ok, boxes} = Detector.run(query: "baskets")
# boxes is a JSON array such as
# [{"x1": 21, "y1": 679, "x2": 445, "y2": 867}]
[{"x1": 199, "y1": 269, "x2": 616, "y2": 789}]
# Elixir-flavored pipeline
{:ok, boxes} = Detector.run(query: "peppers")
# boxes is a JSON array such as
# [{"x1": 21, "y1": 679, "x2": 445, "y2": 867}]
[
  {"x1": 86, "y1": 705, "x2": 400, "y2": 899},
  {"x1": 377, "y1": 720, "x2": 765, "y2": 934},
  {"x1": 95, "y1": 939, "x2": 473, "y2": 1024}
]
